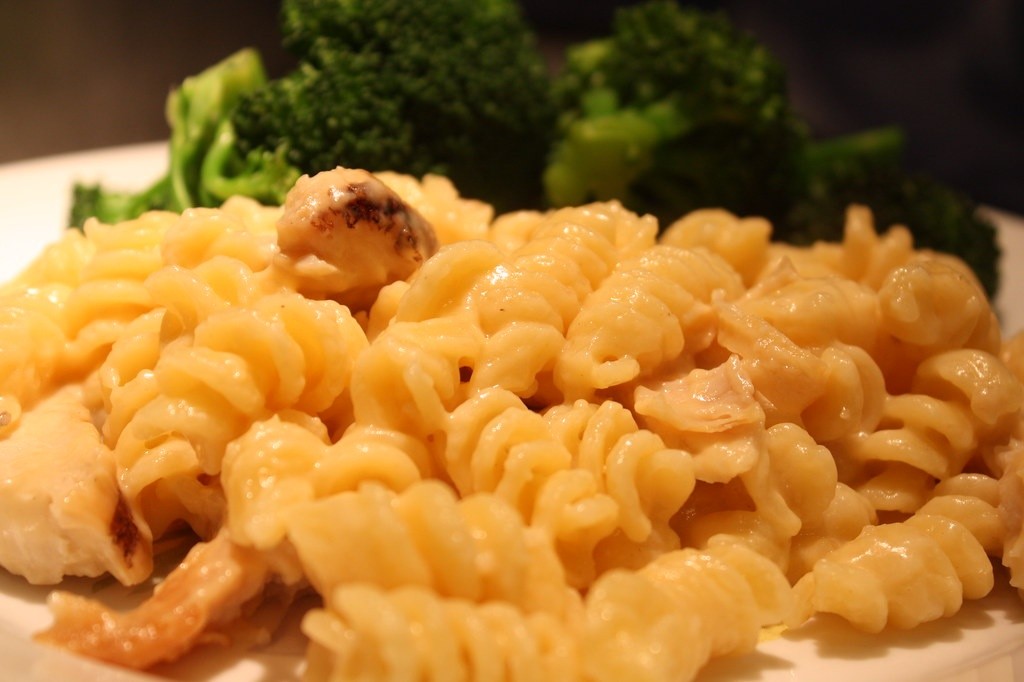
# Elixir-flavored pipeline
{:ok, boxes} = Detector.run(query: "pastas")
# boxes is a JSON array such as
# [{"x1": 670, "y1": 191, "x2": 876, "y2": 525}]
[{"x1": 2, "y1": 168, "x2": 1024, "y2": 682}]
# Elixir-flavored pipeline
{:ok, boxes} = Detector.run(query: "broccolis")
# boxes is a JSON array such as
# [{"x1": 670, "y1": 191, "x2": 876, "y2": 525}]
[{"x1": 66, "y1": 0, "x2": 1024, "y2": 298}]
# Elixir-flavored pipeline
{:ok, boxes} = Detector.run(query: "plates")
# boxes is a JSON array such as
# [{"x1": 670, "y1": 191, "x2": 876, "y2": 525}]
[{"x1": 2, "y1": 140, "x2": 1021, "y2": 682}]
